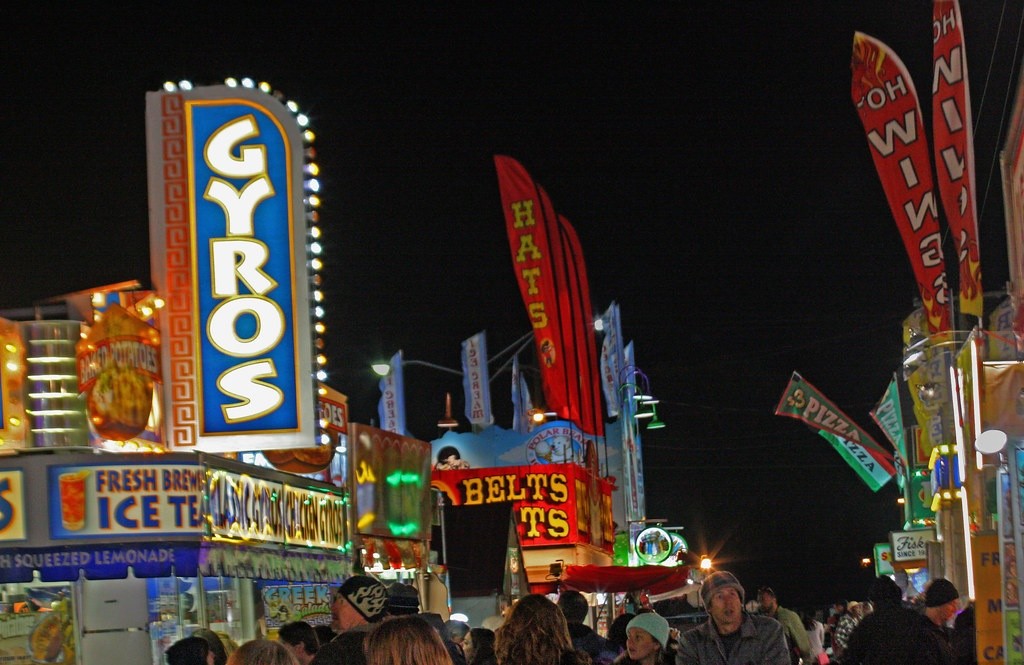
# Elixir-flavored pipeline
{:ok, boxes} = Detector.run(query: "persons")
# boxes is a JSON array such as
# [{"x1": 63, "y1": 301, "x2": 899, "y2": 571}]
[
  {"x1": 676, "y1": 571, "x2": 792, "y2": 665},
  {"x1": 492, "y1": 593, "x2": 592, "y2": 665},
  {"x1": 556, "y1": 590, "x2": 624, "y2": 665},
  {"x1": 368, "y1": 615, "x2": 453, "y2": 665},
  {"x1": 420, "y1": 613, "x2": 497, "y2": 665},
  {"x1": 757, "y1": 587, "x2": 815, "y2": 665},
  {"x1": 384, "y1": 582, "x2": 420, "y2": 623},
  {"x1": 226, "y1": 639, "x2": 289, "y2": 665},
  {"x1": 165, "y1": 628, "x2": 238, "y2": 665},
  {"x1": 805, "y1": 600, "x2": 872, "y2": 665},
  {"x1": 277, "y1": 621, "x2": 319, "y2": 665},
  {"x1": 312, "y1": 575, "x2": 387, "y2": 665},
  {"x1": 845, "y1": 574, "x2": 948, "y2": 665},
  {"x1": 609, "y1": 608, "x2": 679, "y2": 665},
  {"x1": 924, "y1": 578, "x2": 977, "y2": 665}
]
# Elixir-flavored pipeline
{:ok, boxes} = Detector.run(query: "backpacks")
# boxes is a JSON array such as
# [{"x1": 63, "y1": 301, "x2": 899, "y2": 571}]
[{"x1": 950, "y1": 601, "x2": 977, "y2": 664}]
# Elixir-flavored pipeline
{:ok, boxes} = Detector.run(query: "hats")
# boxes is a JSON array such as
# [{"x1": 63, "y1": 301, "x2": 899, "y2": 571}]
[
  {"x1": 846, "y1": 601, "x2": 858, "y2": 610},
  {"x1": 926, "y1": 577, "x2": 959, "y2": 606},
  {"x1": 639, "y1": 595, "x2": 649, "y2": 605},
  {"x1": 337, "y1": 574, "x2": 389, "y2": 623},
  {"x1": 698, "y1": 571, "x2": 744, "y2": 617},
  {"x1": 386, "y1": 583, "x2": 419, "y2": 609},
  {"x1": 869, "y1": 576, "x2": 902, "y2": 600},
  {"x1": 626, "y1": 612, "x2": 669, "y2": 650}
]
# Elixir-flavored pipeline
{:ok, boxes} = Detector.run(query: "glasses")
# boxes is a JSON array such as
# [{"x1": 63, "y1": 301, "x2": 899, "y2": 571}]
[{"x1": 758, "y1": 585, "x2": 775, "y2": 594}]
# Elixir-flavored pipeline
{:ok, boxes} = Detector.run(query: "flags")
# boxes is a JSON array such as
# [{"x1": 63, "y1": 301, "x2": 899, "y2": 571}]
[
  {"x1": 819, "y1": 429, "x2": 897, "y2": 492},
  {"x1": 379, "y1": 350, "x2": 405, "y2": 435},
  {"x1": 462, "y1": 332, "x2": 492, "y2": 428},
  {"x1": 851, "y1": 33, "x2": 953, "y2": 340},
  {"x1": 600, "y1": 301, "x2": 638, "y2": 417},
  {"x1": 493, "y1": 152, "x2": 604, "y2": 435},
  {"x1": 929, "y1": 0, "x2": 985, "y2": 317}
]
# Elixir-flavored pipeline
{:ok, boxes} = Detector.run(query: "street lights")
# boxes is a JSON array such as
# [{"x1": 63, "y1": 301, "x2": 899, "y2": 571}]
[
  {"x1": 695, "y1": 556, "x2": 712, "y2": 615},
  {"x1": 436, "y1": 393, "x2": 459, "y2": 431}
]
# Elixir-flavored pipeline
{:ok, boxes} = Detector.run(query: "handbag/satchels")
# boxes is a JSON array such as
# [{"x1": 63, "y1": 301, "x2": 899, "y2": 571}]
[{"x1": 815, "y1": 653, "x2": 830, "y2": 665}]
